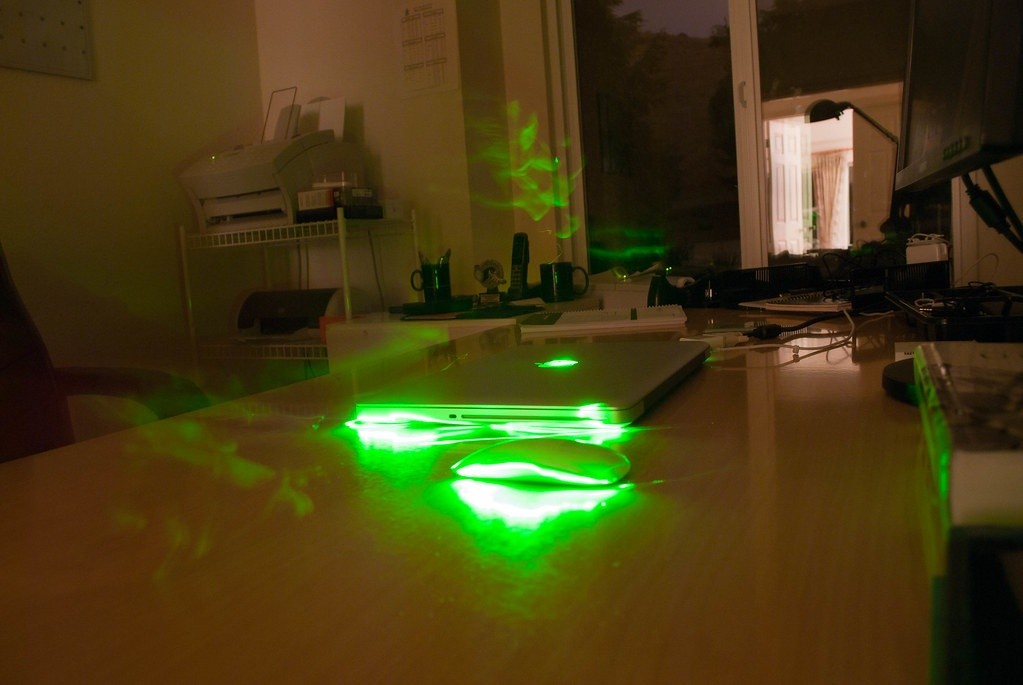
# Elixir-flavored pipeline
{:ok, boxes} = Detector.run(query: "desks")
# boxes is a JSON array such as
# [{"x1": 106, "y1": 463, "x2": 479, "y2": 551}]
[{"x1": 1, "y1": 298, "x2": 936, "y2": 684}]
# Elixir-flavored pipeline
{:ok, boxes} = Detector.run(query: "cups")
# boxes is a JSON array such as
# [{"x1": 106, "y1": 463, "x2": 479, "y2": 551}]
[
  {"x1": 539, "y1": 261, "x2": 588, "y2": 304},
  {"x1": 411, "y1": 265, "x2": 452, "y2": 304}
]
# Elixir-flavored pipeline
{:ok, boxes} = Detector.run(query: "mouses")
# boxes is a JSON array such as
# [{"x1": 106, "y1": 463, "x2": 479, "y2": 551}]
[{"x1": 450, "y1": 437, "x2": 632, "y2": 488}]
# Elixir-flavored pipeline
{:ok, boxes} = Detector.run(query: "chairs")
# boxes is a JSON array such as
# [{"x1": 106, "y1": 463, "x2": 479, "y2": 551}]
[{"x1": 0, "y1": 250, "x2": 212, "y2": 459}]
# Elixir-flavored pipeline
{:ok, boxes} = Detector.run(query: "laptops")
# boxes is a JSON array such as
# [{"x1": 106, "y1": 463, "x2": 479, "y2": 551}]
[{"x1": 356, "y1": 338, "x2": 711, "y2": 429}]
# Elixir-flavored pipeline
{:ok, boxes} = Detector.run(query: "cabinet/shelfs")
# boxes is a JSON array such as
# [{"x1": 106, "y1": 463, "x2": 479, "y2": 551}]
[
  {"x1": 177, "y1": 204, "x2": 424, "y2": 393},
  {"x1": 327, "y1": 297, "x2": 605, "y2": 376}
]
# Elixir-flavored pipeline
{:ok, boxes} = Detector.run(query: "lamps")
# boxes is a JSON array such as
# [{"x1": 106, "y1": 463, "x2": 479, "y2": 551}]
[{"x1": 806, "y1": 97, "x2": 907, "y2": 234}]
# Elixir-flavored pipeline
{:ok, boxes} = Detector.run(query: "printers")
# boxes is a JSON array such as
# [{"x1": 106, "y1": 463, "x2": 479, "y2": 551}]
[
  {"x1": 232, "y1": 285, "x2": 373, "y2": 346},
  {"x1": 178, "y1": 95, "x2": 368, "y2": 237}
]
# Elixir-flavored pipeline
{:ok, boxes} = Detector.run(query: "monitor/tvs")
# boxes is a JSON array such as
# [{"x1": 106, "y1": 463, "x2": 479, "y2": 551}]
[{"x1": 894, "y1": 0, "x2": 1023, "y2": 192}]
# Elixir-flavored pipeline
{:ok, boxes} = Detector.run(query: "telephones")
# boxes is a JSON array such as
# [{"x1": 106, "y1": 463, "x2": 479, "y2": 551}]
[{"x1": 509, "y1": 233, "x2": 529, "y2": 300}]
